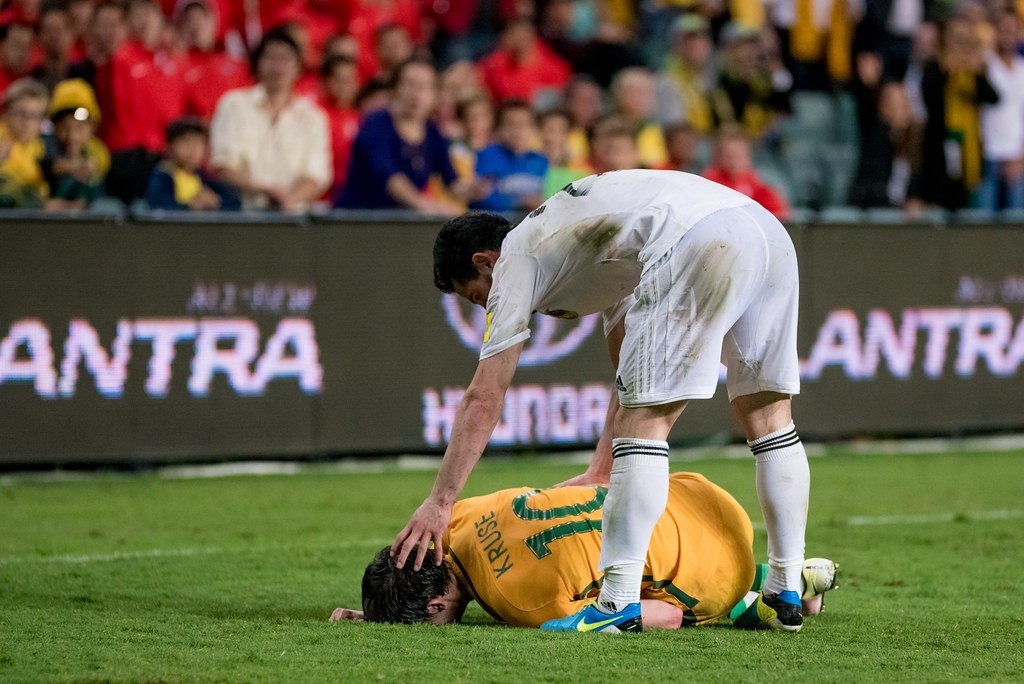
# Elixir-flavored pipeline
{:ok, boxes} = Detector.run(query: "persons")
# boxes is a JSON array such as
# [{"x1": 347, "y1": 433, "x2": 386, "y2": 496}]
[
  {"x1": 0, "y1": 0, "x2": 1024, "y2": 221},
  {"x1": 329, "y1": 472, "x2": 840, "y2": 628},
  {"x1": 389, "y1": 170, "x2": 811, "y2": 635}
]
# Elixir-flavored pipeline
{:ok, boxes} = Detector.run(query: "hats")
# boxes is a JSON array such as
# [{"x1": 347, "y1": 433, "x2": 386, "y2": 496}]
[
  {"x1": 47, "y1": 79, "x2": 102, "y2": 121},
  {"x1": 667, "y1": 13, "x2": 708, "y2": 36},
  {"x1": 722, "y1": 20, "x2": 759, "y2": 40}
]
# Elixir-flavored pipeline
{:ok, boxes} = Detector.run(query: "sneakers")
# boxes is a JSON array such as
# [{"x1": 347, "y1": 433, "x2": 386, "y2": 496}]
[
  {"x1": 733, "y1": 590, "x2": 803, "y2": 632},
  {"x1": 540, "y1": 601, "x2": 643, "y2": 633},
  {"x1": 801, "y1": 558, "x2": 839, "y2": 616}
]
